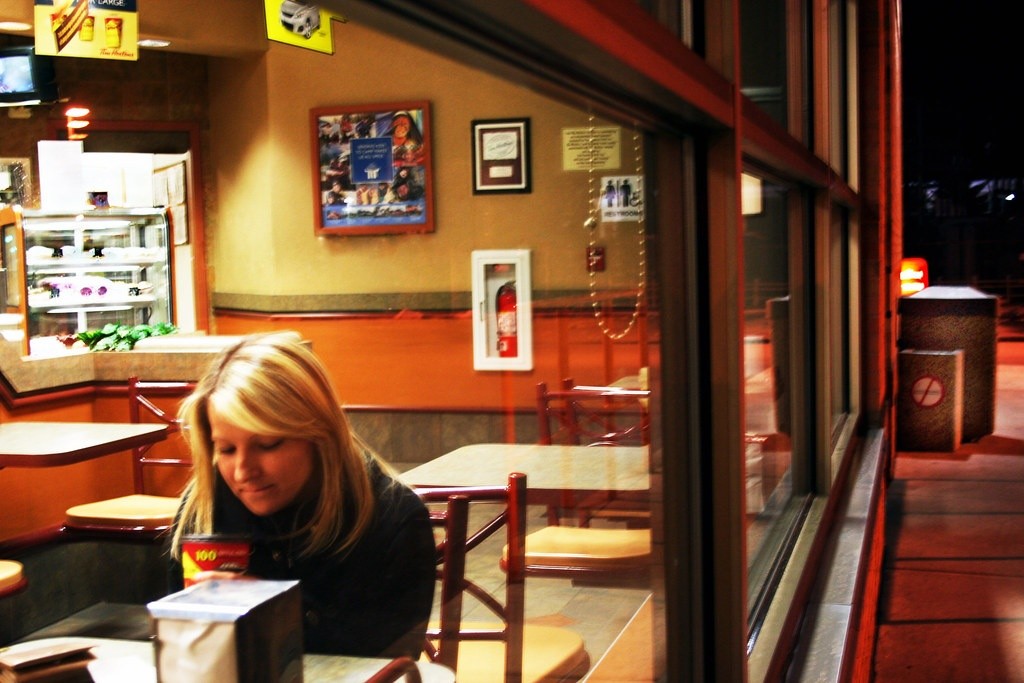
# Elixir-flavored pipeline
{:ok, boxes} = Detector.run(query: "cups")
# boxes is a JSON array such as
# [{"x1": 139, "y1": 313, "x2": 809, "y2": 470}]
[{"x1": 180, "y1": 534, "x2": 253, "y2": 589}]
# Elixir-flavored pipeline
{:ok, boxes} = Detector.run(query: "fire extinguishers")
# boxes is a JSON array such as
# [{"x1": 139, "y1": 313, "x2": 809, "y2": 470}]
[{"x1": 493, "y1": 279, "x2": 518, "y2": 359}]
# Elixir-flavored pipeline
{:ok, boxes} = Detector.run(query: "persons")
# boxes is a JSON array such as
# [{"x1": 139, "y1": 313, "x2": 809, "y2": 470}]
[
  {"x1": 318, "y1": 110, "x2": 422, "y2": 206},
  {"x1": 138, "y1": 334, "x2": 436, "y2": 662}
]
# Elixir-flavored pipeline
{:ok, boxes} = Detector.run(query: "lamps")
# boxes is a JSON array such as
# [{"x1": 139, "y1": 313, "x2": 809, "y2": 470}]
[
  {"x1": 58, "y1": 69, "x2": 91, "y2": 118},
  {"x1": 66, "y1": 128, "x2": 90, "y2": 141},
  {"x1": 66, "y1": 116, "x2": 89, "y2": 129}
]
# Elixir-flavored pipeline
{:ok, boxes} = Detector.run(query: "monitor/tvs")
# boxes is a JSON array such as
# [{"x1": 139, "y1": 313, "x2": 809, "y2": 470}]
[{"x1": 0, "y1": 46, "x2": 59, "y2": 102}]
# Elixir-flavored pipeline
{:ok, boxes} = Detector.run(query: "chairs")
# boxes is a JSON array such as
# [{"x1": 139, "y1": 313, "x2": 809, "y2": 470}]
[
  {"x1": 428, "y1": 491, "x2": 471, "y2": 675},
  {"x1": 0, "y1": 561, "x2": 28, "y2": 599},
  {"x1": 409, "y1": 471, "x2": 591, "y2": 683},
  {"x1": 60, "y1": 376, "x2": 202, "y2": 549},
  {"x1": 537, "y1": 382, "x2": 652, "y2": 577},
  {"x1": 560, "y1": 375, "x2": 651, "y2": 528}
]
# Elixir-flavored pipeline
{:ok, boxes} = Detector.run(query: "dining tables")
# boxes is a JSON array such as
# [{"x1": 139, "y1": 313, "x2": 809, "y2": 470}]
[
  {"x1": 606, "y1": 370, "x2": 776, "y2": 398},
  {"x1": 0, "y1": 637, "x2": 455, "y2": 683},
  {"x1": 0, "y1": 418, "x2": 172, "y2": 465},
  {"x1": 395, "y1": 442, "x2": 652, "y2": 505}
]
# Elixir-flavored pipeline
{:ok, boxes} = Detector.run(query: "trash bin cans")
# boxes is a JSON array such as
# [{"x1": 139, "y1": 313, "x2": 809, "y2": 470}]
[{"x1": 900, "y1": 282, "x2": 1000, "y2": 443}]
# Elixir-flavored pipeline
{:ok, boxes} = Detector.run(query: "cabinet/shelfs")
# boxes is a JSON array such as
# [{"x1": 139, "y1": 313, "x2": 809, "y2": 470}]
[
  {"x1": 0, "y1": 204, "x2": 177, "y2": 357},
  {"x1": 0, "y1": 313, "x2": 311, "y2": 560}
]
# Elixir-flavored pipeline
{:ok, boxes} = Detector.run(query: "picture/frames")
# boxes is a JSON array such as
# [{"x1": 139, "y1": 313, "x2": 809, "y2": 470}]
[{"x1": 308, "y1": 100, "x2": 435, "y2": 234}]
